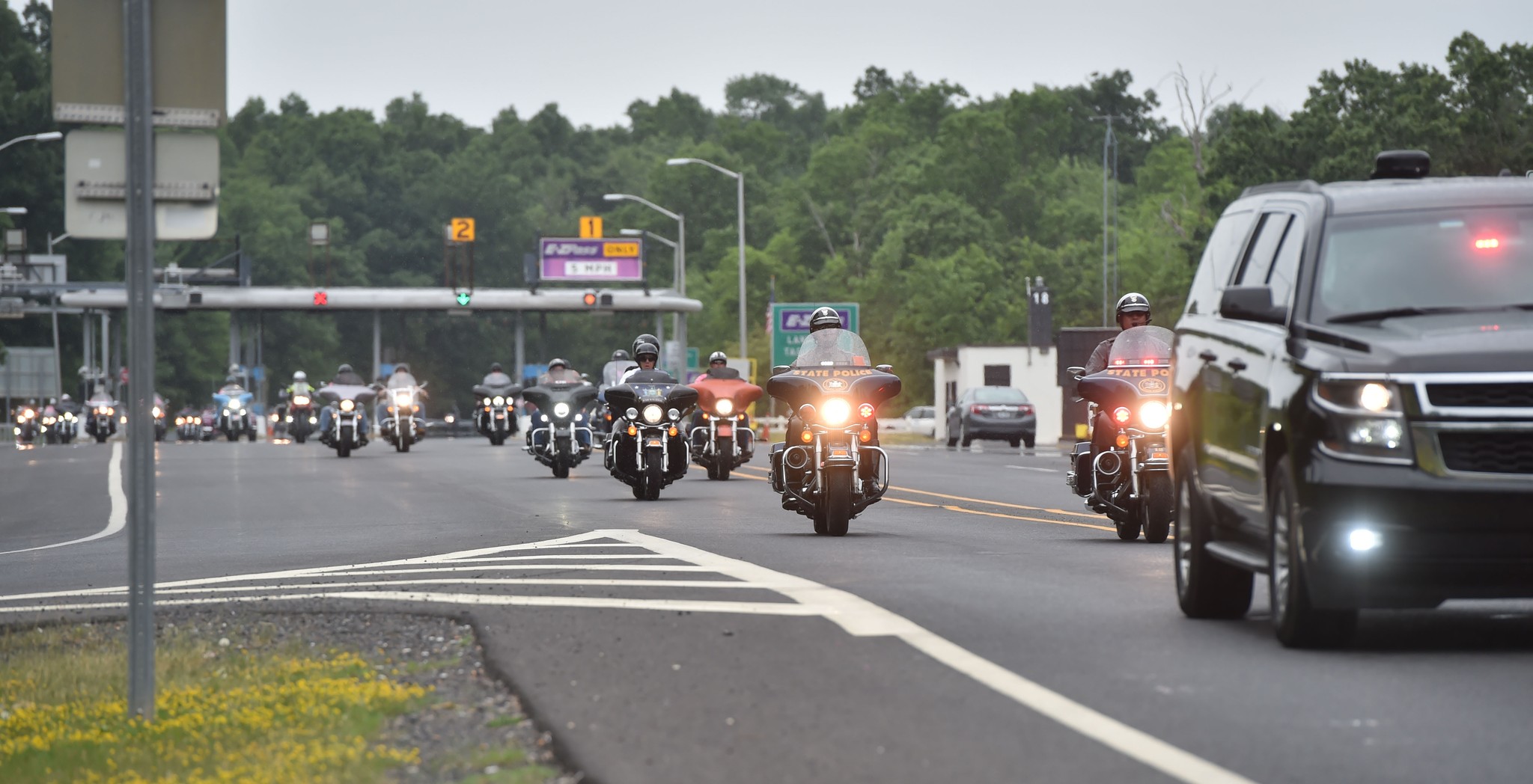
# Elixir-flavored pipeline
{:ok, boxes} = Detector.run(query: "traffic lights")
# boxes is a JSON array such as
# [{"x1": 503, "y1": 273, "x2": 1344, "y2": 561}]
[
  {"x1": 601, "y1": 295, "x2": 612, "y2": 306},
  {"x1": 314, "y1": 291, "x2": 328, "y2": 305},
  {"x1": 584, "y1": 293, "x2": 596, "y2": 305},
  {"x1": 458, "y1": 293, "x2": 470, "y2": 305}
]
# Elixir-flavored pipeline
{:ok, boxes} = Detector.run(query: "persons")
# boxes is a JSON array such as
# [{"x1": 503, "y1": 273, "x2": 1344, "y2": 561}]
[
  {"x1": 19, "y1": 362, "x2": 432, "y2": 441},
  {"x1": 1087, "y1": 293, "x2": 1170, "y2": 506},
  {"x1": 477, "y1": 333, "x2": 753, "y2": 464},
  {"x1": 782, "y1": 306, "x2": 882, "y2": 510}
]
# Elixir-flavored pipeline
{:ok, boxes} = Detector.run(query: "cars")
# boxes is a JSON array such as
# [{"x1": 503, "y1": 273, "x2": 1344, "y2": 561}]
[{"x1": 944, "y1": 386, "x2": 1036, "y2": 448}]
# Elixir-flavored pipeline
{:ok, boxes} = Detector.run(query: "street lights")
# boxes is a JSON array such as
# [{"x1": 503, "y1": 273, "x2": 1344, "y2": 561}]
[
  {"x1": 1, "y1": 206, "x2": 28, "y2": 215},
  {"x1": 1, "y1": 131, "x2": 61, "y2": 153},
  {"x1": 605, "y1": 193, "x2": 687, "y2": 380},
  {"x1": 665, "y1": 156, "x2": 749, "y2": 365}
]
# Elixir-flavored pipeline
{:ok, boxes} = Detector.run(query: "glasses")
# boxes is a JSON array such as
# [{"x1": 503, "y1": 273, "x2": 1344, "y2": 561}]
[
  {"x1": 1124, "y1": 310, "x2": 1145, "y2": 316},
  {"x1": 711, "y1": 359, "x2": 725, "y2": 364},
  {"x1": 816, "y1": 323, "x2": 837, "y2": 331},
  {"x1": 638, "y1": 355, "x2": 656, "y2": 362}
]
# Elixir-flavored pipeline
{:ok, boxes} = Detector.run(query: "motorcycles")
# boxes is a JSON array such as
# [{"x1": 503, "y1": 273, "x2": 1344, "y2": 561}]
[
  {"x1": 178, "y1": 406, "x2": 215, "y2": 438},
  {"x1": 684, "y1": 368, "x2": 763, "y2": 479},
  {"x1": 593, "y1": 361, "x2": 659, "y2": 450},
  {"x1": 1064, "y1": 328, "x2": 1179, "y2": 543},
  {"x1": 153, "y1": 394, "x2": 171, "y2": 440},
  {"x1": 522, "y1": 370, "x2": 598, "y2": 477},
  {"x1": 470, "y1": 372, "x2": 522, "y2": 445},
  {"x1": 17, "y1": 401, "x2": 80, "y2": 444},
  {"x1": 369, "y1": 371, "x2": 431, "y2": 452},
  {"x1": 83, "y1": 392, "x2": 120, "y2": 445},
  {"x1": 767, "y1": 328, "x2": 902, "y2": 537},
  {"x1": 277, "y1": 381, "x2": 320, "y2": 444},
  {"x1": 212, "y1": 385, "x2": 253, "y2": 442},
  {"x1": 319, "y1": 372, "x2": 377, "y2": 457},
  {"x1": 604, "y1": 371, "x2": 699, "y2": 501}
]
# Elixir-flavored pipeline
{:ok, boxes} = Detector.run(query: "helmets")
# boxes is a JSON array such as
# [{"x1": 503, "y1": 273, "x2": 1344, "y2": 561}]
[
  {"x1": 635, "y1": 343, "x2": 658, "y2": 360},
  {"x1": 631, "y1": 334, "x2": 659, "y2": 354},
  {"x1": 394, "y1": 363, "x2": 410, "y2": 373},
  {"x1": 611, "y1": 349, "x2": 631, "y2": 361},
  {"x1": 710, "y1": 351, "x2": 727, "y2": 364},
  {"x1": 548, "y1": 359, "x2": 566, "y2": 370},
  {"x1": 9, "y1": 375, "x2": 263, "y2": 429},
  {"x1": 336, "y1": 364, "x2": 353, "y2": 374},
  {"x1": 810, "y1": 306, "x2": 841, "y2": 336},
  {"x1": 1115, "y1": 293, "x2": 1151, "y2": 322},
  {"x1": 292, "y1": 371, "x2": 308, "y2": 379},
  {"x1": 490, "y1": 363, "x2": 502, "y2": 372}
]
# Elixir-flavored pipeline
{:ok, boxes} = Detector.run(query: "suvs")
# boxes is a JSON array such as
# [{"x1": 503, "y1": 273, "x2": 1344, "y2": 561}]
[{"x1": 1172, "y1": 149, "x2": 1533, "y2": 648}]
